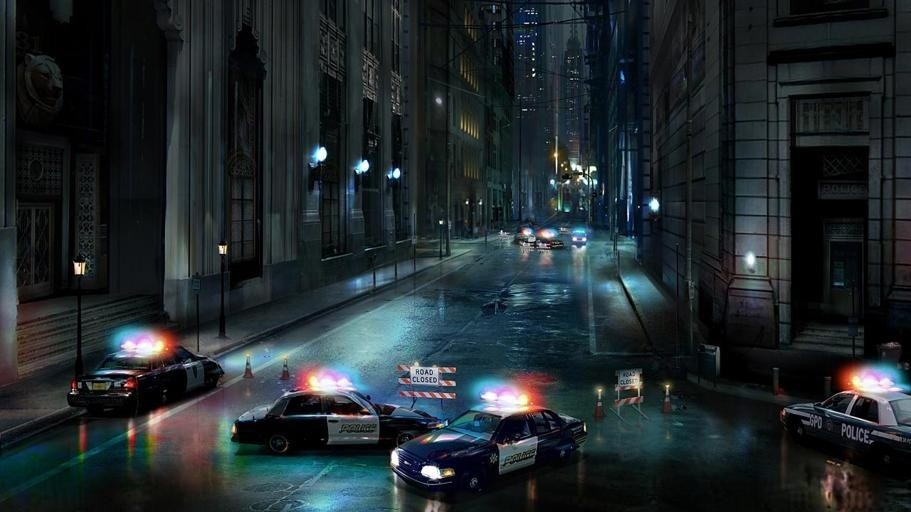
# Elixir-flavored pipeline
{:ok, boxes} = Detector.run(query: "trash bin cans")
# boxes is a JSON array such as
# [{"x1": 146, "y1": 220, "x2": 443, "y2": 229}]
[{"x1": 695, "y1": 342, "x2": 720, "y2": 392}]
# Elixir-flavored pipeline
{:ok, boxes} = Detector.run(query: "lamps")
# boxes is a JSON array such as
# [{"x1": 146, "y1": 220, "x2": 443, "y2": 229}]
[
  {"x1": 308, "y1": 146, "x2": 327, "y2": 194},
  {"x1": 353, "y1": 158, "x2": 370, "y2": 191},
  {"x1": 647, "y1": 195, "x2": 661, "y2": 223},
  {"x1": 386, "y1": 166, "x2": 401, "y2": 194}
]
